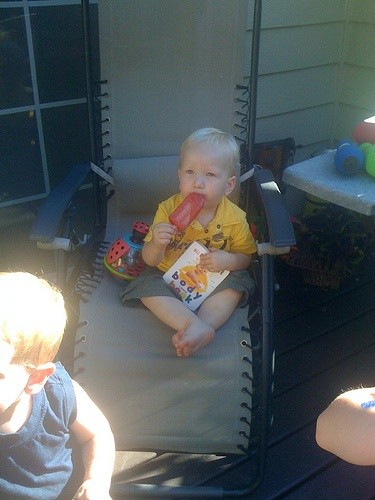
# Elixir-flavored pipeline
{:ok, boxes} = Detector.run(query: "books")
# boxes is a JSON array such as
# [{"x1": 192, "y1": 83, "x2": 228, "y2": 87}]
[{"x1": 162, "y1": 241, "x2": 231, "y2": 311}]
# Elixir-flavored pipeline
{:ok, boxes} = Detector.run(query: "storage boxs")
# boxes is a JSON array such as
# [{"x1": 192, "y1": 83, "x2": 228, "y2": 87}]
[{"x1": 273, "y1": 216, "x2": 368, "y2": 290}]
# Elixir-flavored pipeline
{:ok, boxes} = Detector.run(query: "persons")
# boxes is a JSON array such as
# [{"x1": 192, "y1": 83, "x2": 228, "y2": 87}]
[
  {"x1": 0, "y1": 272, "x2": 116, "y2": 500},
  {"x1": 119, "y1": 127, "x2": 257, "y2": 358}
]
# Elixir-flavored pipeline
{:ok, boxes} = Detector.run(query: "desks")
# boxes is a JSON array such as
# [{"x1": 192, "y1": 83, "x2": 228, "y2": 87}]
[{"x1": 283, "y1": 148, "x2": 375, "y2": 216}]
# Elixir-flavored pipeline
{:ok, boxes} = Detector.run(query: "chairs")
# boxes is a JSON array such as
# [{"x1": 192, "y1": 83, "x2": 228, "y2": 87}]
[{"x1": 29, "y1": 0, "x2": 297, "y2": 500}]
